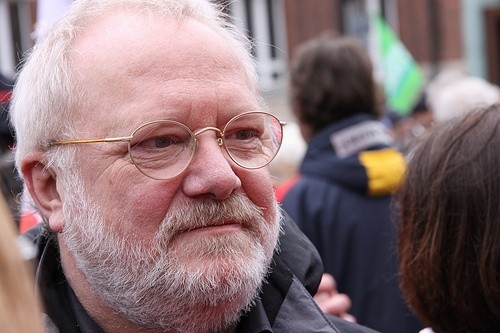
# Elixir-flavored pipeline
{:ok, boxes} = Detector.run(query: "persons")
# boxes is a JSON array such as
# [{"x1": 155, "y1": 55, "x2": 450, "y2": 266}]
[
  {"x1": 312, "y1": 272, "x2": 357, "y2": 323},
  {"x1": 273, "y1": 36, "x2": 429, "y2": 333},
  {"x1": 8, "y1": 1, "x2": 377, "y2": 333},
  {"x1": 398, "y1": 101, "x2": 500, "y2": 333},
  {"x1": 388, "y1": 66, "x2": 500, "y2": 151}
]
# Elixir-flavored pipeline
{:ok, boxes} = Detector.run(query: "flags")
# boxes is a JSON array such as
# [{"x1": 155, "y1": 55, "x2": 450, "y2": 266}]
[{"x1": 368, "y1": 12, "x2": 425, "y2": 117}]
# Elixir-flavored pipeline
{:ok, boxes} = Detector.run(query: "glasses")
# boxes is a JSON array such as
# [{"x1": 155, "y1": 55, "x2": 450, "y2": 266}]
[{"x1": 29, "y1": 111, "x2": 287, "y2": 182}]
